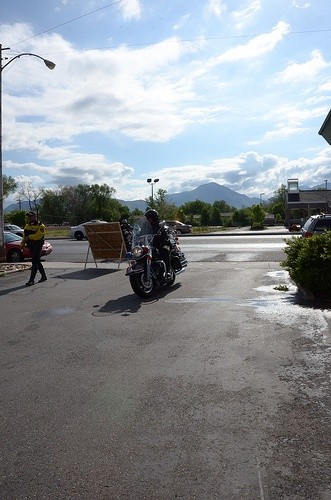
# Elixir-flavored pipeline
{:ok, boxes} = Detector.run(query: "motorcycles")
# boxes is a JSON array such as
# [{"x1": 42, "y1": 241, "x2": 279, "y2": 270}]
[{"x1": 122, "y1": 219, "x2": 188, "y2": 299}]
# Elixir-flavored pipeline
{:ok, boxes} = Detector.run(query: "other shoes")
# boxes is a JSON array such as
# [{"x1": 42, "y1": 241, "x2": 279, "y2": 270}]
[
  {"x1": 38, "y1": 277, "x2": 47, "y2": 283},
  {"x1": 26, "y1": 280, "x2": 35, "y2": 285}
]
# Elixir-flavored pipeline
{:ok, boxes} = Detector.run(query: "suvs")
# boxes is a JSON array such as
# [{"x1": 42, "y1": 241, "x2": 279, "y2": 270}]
[{"x1": 300, "y1": 212, "x2": 331, "y2": 238}]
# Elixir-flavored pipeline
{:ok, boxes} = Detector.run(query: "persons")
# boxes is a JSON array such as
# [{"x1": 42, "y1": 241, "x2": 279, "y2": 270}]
[
  {"x1": 121, "y1": 219, "x2": 131, "y2": 231},
  {"x1": 144, "y1": 209, "x2": 171, "y2": 275},
  {"x1": 19, "y1": 212, "x2": 47, "y2": 286}
]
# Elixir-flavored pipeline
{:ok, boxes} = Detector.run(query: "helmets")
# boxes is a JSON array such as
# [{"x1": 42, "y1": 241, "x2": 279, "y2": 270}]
[{"x1": 145, "y1": 209, "x2": 159, "y2": 226}]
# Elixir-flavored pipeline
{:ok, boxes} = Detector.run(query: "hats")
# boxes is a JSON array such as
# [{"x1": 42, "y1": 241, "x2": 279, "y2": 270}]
[{"x1": 27, "y1": 212, "x2": 36, "y2": 216}]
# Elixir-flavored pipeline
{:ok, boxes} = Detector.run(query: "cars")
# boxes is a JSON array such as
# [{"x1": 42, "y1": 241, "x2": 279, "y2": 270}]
[
  {"x1": 69, "y1": 220, "x2": 108, "y2": 240},
  {"x1": 289, "y1": 224, "x2": 301, "y2": 231},
  {"x1": 3, "y1": 225, "x2": 53, "y2": 263},
  {"x1": 160, "y1": 220, "x2": 193, "y2": 234}
]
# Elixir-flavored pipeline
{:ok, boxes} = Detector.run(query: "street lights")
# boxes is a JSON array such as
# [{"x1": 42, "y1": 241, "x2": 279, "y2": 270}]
[
  {"x1": 260, "y1": 193, "x2": 265, "y2": 206},
  {"x1": 324, "y1": 180, "x2": 328, "y2": 212},
  {"x1": 147, "y1": 178, "x2": 159, "y2": 209},
  {"x1": 0, "y1": 44, "x2": 56, "y2": 263}
]
